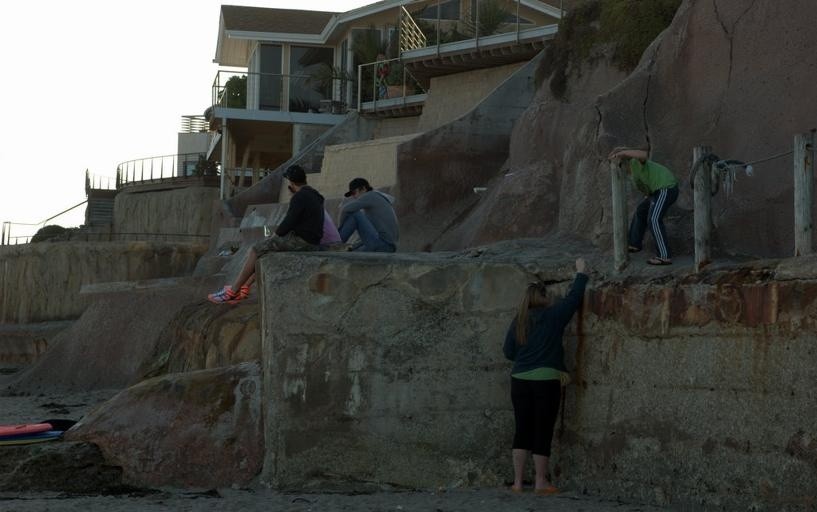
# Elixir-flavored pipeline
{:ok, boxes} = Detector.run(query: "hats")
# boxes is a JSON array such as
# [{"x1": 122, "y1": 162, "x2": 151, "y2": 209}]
[
  {"x1": 344, "y1": 177, "x2": 369, "y2": 198},
  {"x1": 283, "y1": 165, "x2": 306, "y2": 181}
]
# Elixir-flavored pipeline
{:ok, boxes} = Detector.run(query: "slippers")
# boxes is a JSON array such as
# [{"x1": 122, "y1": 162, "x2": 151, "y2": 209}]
[
  {"x1": 646, "y1": 256, "x2": 673, "y2": 265},
  {"x1": 511, "y1": 485, "x2": 559, "y2": 494}
]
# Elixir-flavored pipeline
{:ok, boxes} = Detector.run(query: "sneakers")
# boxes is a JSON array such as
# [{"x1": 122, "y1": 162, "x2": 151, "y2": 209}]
[
  {"x1": 207, "y1": 285, "x2": 236, "y2": 304},
  {"x1": 228, "y1": 285, "x2": 250, "y2": 305}
]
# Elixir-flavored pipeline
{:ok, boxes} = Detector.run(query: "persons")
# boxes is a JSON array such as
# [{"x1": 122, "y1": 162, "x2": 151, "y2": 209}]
[
  {"x1": 375, "y1": 52, "x2": 389, "y2": 100},
  {"x1": 499, "y1": 256, "x2": 589, "y2": 497},
  {"x1": 287, "y1": 186, "x2": 342, "y2": 251},
  {"x1": 334, "y1": 176, "x2": 400, "y2": 254},
  {"x1": 607, "y1": 145, "x2": 679, "y2": 266},
  {"x1": 206, "y1": 164, "x2": 324, "y2": 306}
]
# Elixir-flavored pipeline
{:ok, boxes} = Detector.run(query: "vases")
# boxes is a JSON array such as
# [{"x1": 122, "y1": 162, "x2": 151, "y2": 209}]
[{"x1": 386, "y1": 85, "x2": 416, "y2": 98}]
